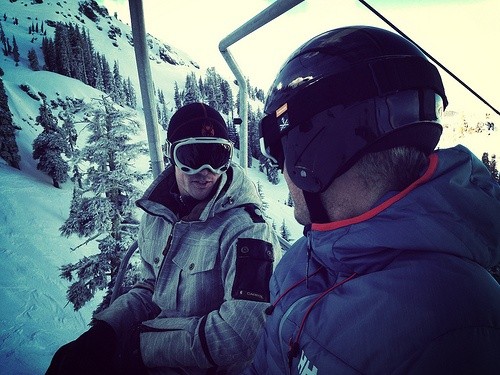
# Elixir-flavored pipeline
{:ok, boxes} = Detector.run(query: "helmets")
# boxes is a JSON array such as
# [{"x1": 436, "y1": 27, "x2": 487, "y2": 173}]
[{"x1": 263, "y1": 24, "x2": 446, "y2": 189}]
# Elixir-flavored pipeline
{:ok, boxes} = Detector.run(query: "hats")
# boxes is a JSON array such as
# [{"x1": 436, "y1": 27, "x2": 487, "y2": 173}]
[{"x1": 164, "y1": 103, "x2": 229, "y2": 142}]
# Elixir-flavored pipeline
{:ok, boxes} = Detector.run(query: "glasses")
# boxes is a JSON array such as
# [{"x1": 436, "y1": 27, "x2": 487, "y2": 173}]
[
  {"x1": 259, "y1": 112, "x2": 287, "y2": 172},
  {"x1": 164, "y1": 139, "x2": 235, "y2": 174}
]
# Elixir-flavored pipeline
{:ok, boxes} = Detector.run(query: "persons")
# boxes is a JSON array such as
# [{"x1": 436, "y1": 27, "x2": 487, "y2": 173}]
[
  {"x1": 42, "y1": 100, "x2": 282, "y2": 375},
  {"x1": 246, "y1": 27, "x2": 500, "y2": 375}
]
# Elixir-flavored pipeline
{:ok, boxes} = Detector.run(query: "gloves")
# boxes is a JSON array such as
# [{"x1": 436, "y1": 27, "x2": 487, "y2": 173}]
[{"x1": 75, "y1": 322, "x2": 124, "y2": 372}]
